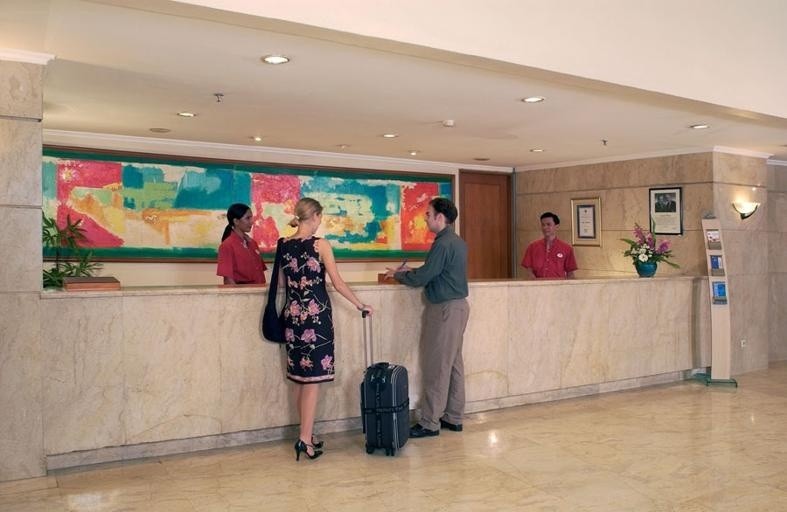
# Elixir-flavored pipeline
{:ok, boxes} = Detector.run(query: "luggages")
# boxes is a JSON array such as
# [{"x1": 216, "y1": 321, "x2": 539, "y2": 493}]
[{"x1": 358, "y1": 310, "x2": 411, "y2": 457}]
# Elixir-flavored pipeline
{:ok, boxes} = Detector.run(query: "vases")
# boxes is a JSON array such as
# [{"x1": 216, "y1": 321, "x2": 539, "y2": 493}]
[{"x1": 635, "y1": 262, "x2": 658, "y2": 277}]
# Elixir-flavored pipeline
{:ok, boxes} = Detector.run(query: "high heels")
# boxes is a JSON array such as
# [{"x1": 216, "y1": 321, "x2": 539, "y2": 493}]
[{"x1": 294, "y1": 430, "x2": 327, "y2": 462}]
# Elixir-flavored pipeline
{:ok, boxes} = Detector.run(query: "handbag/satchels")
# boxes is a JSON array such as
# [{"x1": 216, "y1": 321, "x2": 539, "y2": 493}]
[{"x1": 259, "y1": 305, "x2": 296, "y2": 343}]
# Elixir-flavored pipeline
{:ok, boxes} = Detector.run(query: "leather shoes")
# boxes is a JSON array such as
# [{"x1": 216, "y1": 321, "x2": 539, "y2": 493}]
[
  {"x1": 406, "y1": 420, "x2": 440, "y2": 438},
  {"x1": 440, "y1": 418, "x2": 463, "y2": 432}
]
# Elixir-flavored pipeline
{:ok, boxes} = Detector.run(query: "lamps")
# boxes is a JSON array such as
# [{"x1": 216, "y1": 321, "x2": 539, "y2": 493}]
[{"x1": 730, "y1": 200, "x2": 761, "y2": 220}]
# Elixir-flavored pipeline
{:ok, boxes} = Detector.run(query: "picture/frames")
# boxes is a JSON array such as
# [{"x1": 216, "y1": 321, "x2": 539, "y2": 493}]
[
  {"x1": 41, "y1": 143, "x2": 456, "y2": 267},
  {"x1": 570, "y1": 197, "x2": 604, "y2": 248},
  {"x1": 648, "y1": 188, "x2": 684, "y2": 236}
]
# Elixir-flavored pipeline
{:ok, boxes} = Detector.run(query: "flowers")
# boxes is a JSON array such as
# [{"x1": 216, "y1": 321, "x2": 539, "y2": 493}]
[{"x1": 619, "y1": 218, "x2": 681, "y2": 270}]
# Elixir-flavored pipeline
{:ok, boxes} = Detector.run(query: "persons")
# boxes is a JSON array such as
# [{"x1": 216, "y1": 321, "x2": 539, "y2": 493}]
[
  {"x1": 382, "y1": 197, "x2": 475, "y2": 438},
  {"x1": 517, "y1": 211, "x2": 579, "y2": 278},
  {"x1": 271, "y1": 197, "x2": 373, "y2": 461},
  {"x1": 215, "y1": 201, "x2": 267, "y2": 285},
  {"x1": 654, "y1": 192, "x2": 676, "y2": 212}
]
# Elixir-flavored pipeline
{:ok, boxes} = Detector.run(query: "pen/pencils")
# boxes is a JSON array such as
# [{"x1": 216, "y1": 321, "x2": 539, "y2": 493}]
[{"x1": 400, "y1": 258, "x2": 407, "y2": 269}]
